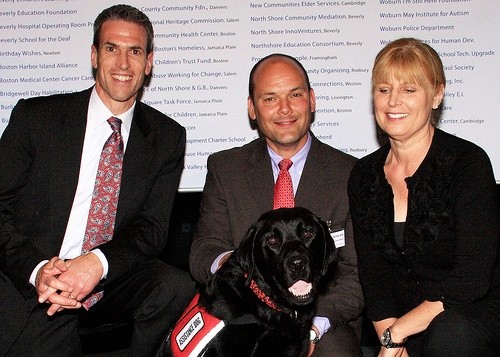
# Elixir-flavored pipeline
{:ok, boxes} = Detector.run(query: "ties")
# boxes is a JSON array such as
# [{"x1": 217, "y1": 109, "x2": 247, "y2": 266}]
[
  {"x1": 273, "y1": 158, "x2": 296, "y2": 209},
  {"x1": 82, "y1": 116, "x2": 123, "y2": 255}
]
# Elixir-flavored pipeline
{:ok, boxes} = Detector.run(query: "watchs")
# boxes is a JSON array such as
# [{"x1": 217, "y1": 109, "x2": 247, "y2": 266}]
[
  {"x1": 309, "y1": 329, "x2": 319, "y2": 344},
  {"x1": 380, "y1": 329, "x2": 405, "y2": 348}
]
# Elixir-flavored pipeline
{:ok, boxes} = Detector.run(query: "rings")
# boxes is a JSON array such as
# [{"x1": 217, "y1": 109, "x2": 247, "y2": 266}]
[{"x1": 70, "y1": 295, "x2": 75, "y2": 299}]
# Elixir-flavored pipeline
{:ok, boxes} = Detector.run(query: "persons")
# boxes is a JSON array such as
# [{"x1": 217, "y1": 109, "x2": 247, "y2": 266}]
[
  {"x1": 0, "y1": 4, "x2": 197, "y2": 357},
  {"x1": 348, "y1": 38, "x2": 499, "y2": 357},
  {"x1": 188, "y1": 52, "x2": 367, "y2": 357}
]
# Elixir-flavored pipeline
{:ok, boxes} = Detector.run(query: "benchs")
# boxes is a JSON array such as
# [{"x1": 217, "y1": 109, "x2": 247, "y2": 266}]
[{"x1": 161, "y1": 194, "x2": 204, "y2": 274}]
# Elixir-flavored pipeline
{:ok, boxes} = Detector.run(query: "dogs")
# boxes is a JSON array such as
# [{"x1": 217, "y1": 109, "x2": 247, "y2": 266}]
[{"x1": 156, "y1": 204, "x2": 341, "y2": 357}]
